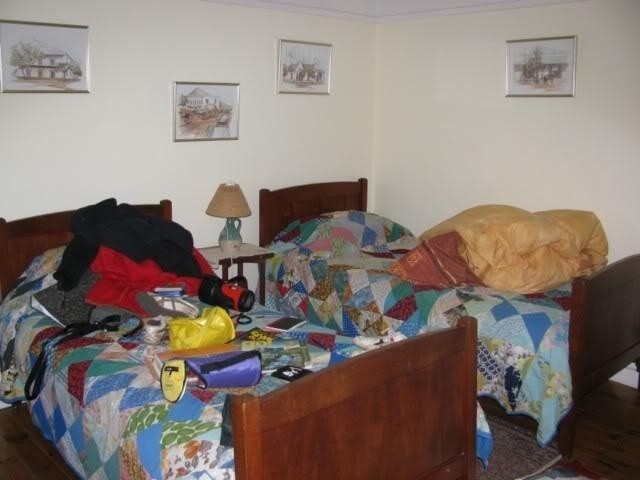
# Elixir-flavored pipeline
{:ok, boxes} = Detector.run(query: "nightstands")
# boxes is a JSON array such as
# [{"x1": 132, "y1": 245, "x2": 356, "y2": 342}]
[{"x1": 198, "y1": 242, "x2": 276, "y2": 306}]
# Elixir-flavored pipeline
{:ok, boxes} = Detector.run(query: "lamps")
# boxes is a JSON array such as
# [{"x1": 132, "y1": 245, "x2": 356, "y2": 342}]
[{"x1": 206, "y1": 182, "x2": 252, "y2": 254}]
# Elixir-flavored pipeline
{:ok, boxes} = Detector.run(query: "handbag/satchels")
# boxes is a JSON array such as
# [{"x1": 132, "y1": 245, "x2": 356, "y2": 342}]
[
  {"x1": 159, "y1": 349, "x2": 263, "y2": 405},
  {"x1": 139, "y1": 290, "x2": 199, "y2": 344},
  {"x1": 167, "y1": 306, "x2": 236, "y2": 349}
]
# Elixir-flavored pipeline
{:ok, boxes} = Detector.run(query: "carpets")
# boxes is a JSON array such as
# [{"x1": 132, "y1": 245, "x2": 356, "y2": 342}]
[{"x1": 475, "y1": 413, "x2": 562, "y2": 480}]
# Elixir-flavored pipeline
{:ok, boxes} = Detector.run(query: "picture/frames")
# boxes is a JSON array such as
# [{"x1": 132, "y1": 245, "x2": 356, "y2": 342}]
[
  {"x1": 277, "y1": 39, "x2": 332, "y2": 93},
  {"x1": 505, "y1": 36, "x2": 575, "y2": 96},
  {"x1": 0, "y1": 20, "x2": 90, "y2": 92},
  {"x1": 172, "y1": 80, "x2": 240, "y2": 142}
]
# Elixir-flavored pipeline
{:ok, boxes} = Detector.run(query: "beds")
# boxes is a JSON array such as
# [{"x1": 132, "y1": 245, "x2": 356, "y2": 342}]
[
  {"x1": 259, "y1": 178, "x2": 640, "y2": 458},
  {"x1": 0, "y1": 198, "x2": 478, "y2": 480}
]
